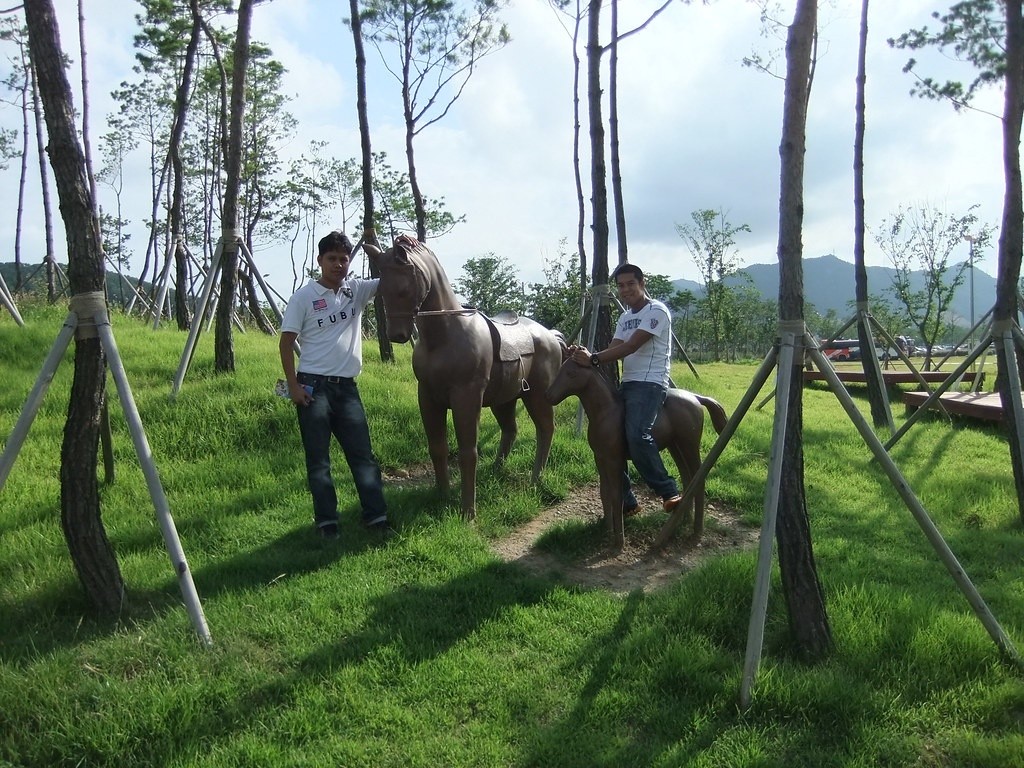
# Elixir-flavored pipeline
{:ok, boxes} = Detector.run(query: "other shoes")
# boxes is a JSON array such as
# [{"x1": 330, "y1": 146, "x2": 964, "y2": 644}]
[
  {"x1": 320, "y1": 523, "x2": 340, "y2": 542},
  {"x1": 368, "y1": 520, "x2": 390, "y2": 533},
  {"x1": 664, "y1": 495, "x2": 682, "y2": 513},
  {"x1": 624, "y1": 505, "x2": 642, "y2": 518}
]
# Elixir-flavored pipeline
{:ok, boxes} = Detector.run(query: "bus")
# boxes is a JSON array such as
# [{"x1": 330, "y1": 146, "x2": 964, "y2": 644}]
[
  {"x1": 820, "y1": 335, "x2": 909, "y2": 363},
  {"x1": 904, "y1": 335, "x2": 916, "y2": 358}
]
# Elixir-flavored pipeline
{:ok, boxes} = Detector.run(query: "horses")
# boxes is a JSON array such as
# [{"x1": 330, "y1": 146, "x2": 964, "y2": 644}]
[{"x1": 361, "y1": 234, "x2": 729, "y2": 550}]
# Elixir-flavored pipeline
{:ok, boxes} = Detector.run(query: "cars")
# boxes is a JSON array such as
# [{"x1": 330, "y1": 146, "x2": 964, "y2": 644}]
[{"x1": 914, "y1": 341, "x2": 997, "y2": 357}]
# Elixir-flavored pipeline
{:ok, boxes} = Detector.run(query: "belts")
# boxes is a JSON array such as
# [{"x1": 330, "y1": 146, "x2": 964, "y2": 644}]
[{"x1": 298, "y1": 372, "x2": 354, "y2": 384}]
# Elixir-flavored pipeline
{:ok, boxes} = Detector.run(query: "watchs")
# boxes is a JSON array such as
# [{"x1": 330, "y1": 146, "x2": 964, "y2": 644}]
[{"x1": 589, "y1": 353, "x2": 601, "y2": 368}]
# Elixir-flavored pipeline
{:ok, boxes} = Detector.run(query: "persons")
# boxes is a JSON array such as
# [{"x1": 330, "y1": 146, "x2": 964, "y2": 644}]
[
  {"x1": 562, "y1": 264, "x2": 683, "y2": 517},
  {"x1": 278, "y1": 230, "x2": 419, "y2": 540}
]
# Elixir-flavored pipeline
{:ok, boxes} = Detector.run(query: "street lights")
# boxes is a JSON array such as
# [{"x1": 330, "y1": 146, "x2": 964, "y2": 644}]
[{"x1": 965, "y1": 234, "x2": 976, "y2": 372}]
